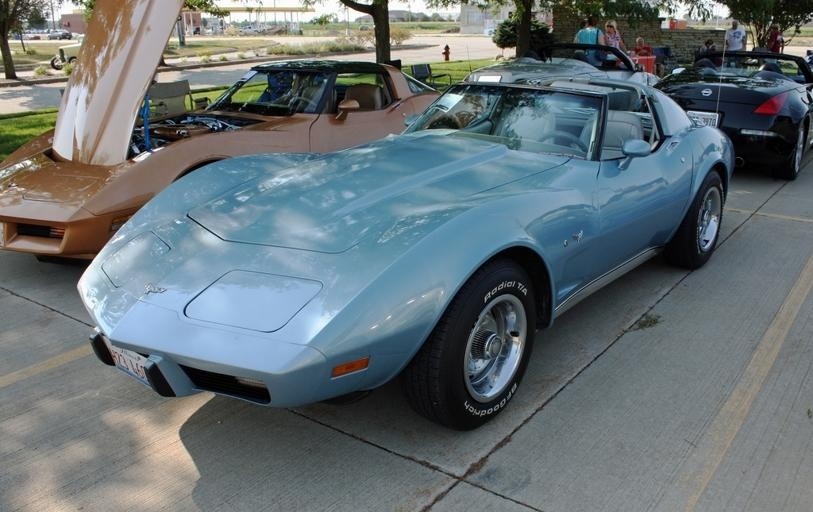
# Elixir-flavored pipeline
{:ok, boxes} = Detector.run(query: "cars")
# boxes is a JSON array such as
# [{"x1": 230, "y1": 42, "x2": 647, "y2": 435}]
[
  {"x1": 8, "y1": 29, "x2": 71, "y2": 41},
  {"x1": 50, "y1": 33, "x2": 85, "y2": 72},
  {"x1": 236, "y1": 24, "x2": 272, "y2": 35}
]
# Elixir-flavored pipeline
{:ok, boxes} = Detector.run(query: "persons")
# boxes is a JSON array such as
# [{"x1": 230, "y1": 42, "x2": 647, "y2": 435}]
[
  {"x1": 631, "y1": 36, "x2": 651, "y2": 57},
  {"x1": 698, "y1": 38, "x2": 716, "y2": 53},
  {"x1": 766, "y1": 22, "x2": 784, "y2": 65},
  {"x1": 573, "y1": 12, "x2": 606, "y2": 69},
  {"x1": 601, "y1": 19, "x2": 626, "y2": 58},
  {"x1": 724, "y1": 21, "x2": 748, "y2": 70},
  {"x1": 750, "y1": 40, "x2": 766, "y2": 53}
]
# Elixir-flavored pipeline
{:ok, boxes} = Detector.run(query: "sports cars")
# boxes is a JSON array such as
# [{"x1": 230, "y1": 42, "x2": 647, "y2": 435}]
[
  {"x1": 76, "y1": 71, "x2": 735, "y2": 428},
  {"x1": 0, "y1": 1, "x2": 488, "y2": 265}
]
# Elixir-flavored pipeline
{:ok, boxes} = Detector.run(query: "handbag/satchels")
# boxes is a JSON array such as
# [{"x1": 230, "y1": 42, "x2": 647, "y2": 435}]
[{"x1": 594, "y1": 48, "x2": 608, "y2": 61}]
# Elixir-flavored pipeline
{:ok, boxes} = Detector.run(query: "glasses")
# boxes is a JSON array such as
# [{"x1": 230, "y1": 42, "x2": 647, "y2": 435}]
[{"x1": 607, "y1": 24, "x2": 613, "y2": 28}]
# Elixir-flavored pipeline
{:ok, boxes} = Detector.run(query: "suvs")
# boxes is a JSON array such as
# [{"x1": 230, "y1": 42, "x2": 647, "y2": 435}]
[{"x1": 193, "y1": 27, "x2": 200, "y2": 35}]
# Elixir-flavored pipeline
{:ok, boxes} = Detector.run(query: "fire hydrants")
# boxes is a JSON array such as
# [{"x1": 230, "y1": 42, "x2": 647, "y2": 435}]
[{"x1": 441, "y1": 44, "x2": 450, "y2": 61}]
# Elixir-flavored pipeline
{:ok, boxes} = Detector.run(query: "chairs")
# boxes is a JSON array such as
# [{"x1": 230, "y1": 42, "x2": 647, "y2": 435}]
[
  {"x1": 135, "y1": 78, "x2": 211, "y2": 128},
  {"x1": 653, "y1": 45, "x2": 679, "y2": 74},
  {"x1": 499, "y1": 106, "x2": 556, "y2": 143},
  {"x1": 295, "y1": 86, "x2": 337, "y2": 113},
  {"x1": 579, "y1": 109, "x2": 643, "y2": 163},
  {"x1": 410, "y1": 63, "x2": 451, "y2": 90},
  {"x1": 757, "y1": 62, "x2": 783, "y2": 73},
  {"x1": 342, "y1": 83, "x2": 383, "y2": 111},
  {"x1": 695, "y1": 57, "x2": 717, "y2": 70}
]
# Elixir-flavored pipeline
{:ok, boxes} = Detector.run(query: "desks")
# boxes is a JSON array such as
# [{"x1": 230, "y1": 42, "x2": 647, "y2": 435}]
[{"x1": 631, "y1": 55, "x2": 655, "y2": 74}]
